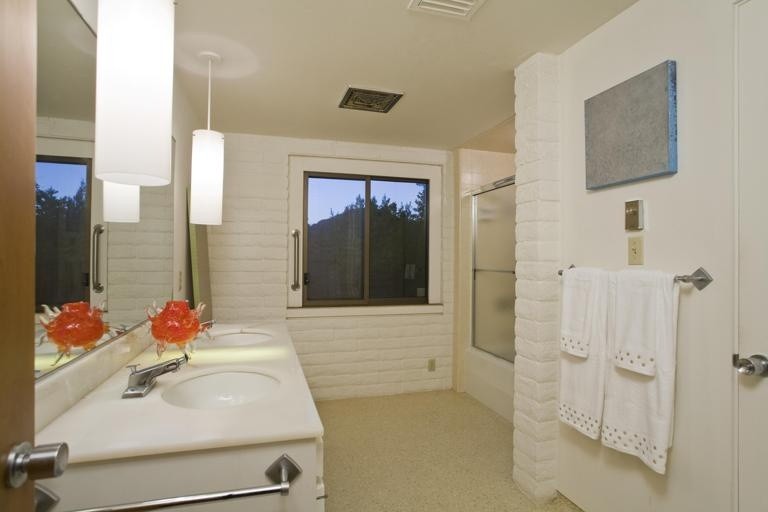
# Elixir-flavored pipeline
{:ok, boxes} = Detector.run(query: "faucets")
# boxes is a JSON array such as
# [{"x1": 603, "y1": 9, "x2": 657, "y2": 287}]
[
  {"x1": 122, "y1": 353, "x2": 188, "y2": 397},
  {"x1": 201, "y1": 319, "x2": 217, "y2": 329}
]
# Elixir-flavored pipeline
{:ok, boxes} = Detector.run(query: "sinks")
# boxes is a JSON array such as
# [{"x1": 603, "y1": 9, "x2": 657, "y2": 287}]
[
  {"x1": 199, "y1": 329, "x2": 274, "y2": 348},
  {"x1": 161, "y1": 367, "x2": 282, "y2": 408}
]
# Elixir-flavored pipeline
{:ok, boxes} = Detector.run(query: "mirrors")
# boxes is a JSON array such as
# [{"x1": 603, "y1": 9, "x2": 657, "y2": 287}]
[{"x1": 33, "y1": 0, "x2": 175, "y2": 423}]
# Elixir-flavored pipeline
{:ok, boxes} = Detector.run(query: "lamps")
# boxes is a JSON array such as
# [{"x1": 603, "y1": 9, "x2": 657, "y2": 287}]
[
  {"x1": 102, "y1": 181, "x2": 142, "y2": 224},
  {"x1": 187, "y1": 50, "x2": 226, "y2": 227},
  {"x1": 92, "y1": 0, "x2": 178, "y2": 186}
]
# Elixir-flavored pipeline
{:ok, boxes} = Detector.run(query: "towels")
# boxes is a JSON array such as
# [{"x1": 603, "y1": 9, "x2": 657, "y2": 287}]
[{"x1": 556, "y1": 264, "x2": 682, "y2": 479}]
